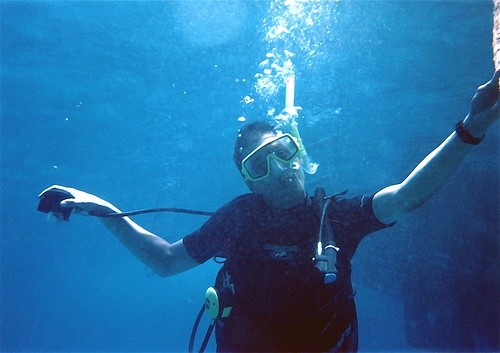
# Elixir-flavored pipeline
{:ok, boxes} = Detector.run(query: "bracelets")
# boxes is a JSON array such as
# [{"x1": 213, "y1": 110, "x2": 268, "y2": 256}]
[{"x1": 455, "y1": 120, "x2": 486, "y2": 145}]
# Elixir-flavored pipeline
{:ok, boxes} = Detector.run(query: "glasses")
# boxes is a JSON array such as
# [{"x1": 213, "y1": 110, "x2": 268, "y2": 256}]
[{"x1": 241, "y1": 133, "x2": 299, "y2": 181}]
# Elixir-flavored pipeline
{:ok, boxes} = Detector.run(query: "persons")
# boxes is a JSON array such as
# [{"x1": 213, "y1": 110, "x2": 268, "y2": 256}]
[{"x1": 39, "y1": 66, "x2": 500, "y2": 353}]
